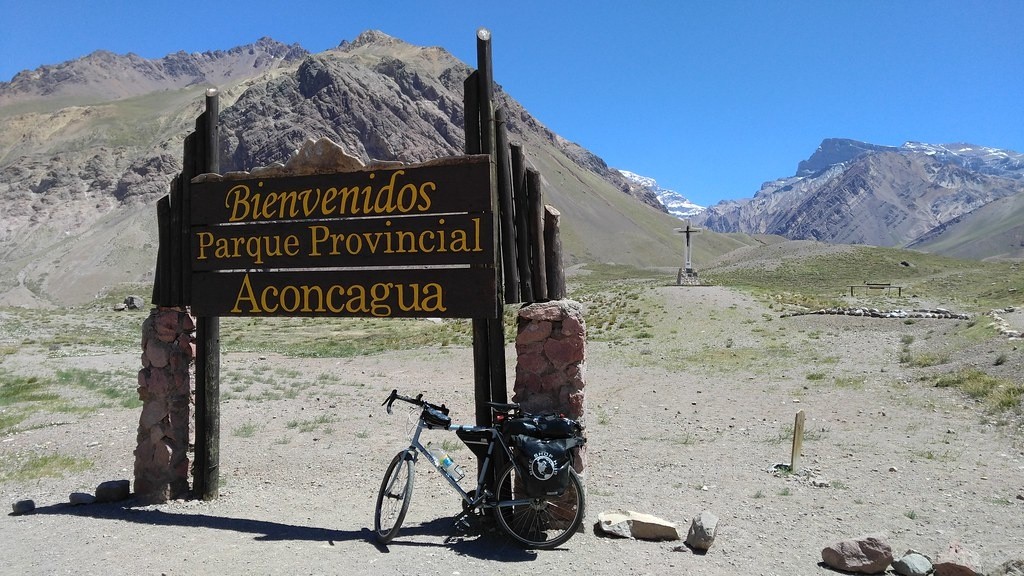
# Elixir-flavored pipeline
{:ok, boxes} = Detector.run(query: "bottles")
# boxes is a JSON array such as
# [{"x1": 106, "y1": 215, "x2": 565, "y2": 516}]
[{"x1": 427, "y1": 441, "x2": 464, "y2": 481}]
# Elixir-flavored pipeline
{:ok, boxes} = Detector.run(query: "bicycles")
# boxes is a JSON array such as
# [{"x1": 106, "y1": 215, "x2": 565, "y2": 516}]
[{"x1": 375, "y1": 388, "x2": 584, "y2": 550}]
